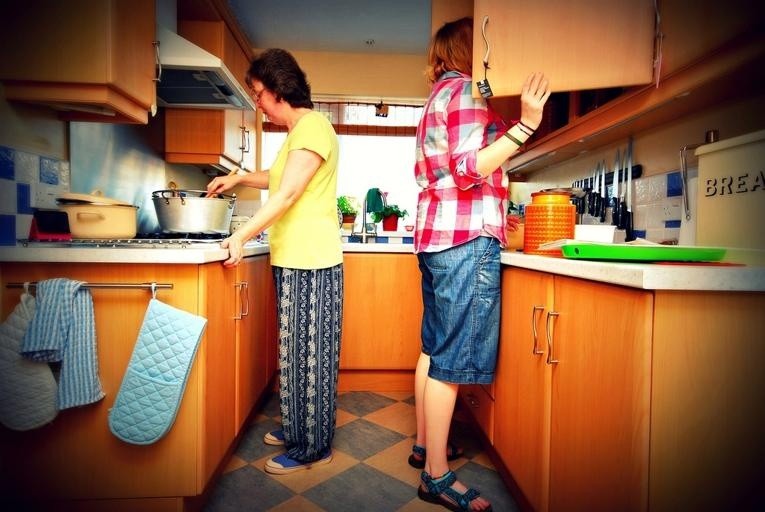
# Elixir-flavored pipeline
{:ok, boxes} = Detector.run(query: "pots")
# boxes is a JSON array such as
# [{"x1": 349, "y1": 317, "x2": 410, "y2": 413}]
[
  {"x1": 153, "y1": 190, "x2": 236, "y2": 235},
  {"x1": 54, "y1": 189, "x2": 140, "y2": 240}
]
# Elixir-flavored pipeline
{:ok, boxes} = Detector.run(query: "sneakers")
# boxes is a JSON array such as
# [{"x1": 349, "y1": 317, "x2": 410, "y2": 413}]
[
  {"x1": 264, "y1": 448, "x2": 332, "y2": 474},
  {"x1": 264, "y1": 430, "x2": 285, "y2": 445}
]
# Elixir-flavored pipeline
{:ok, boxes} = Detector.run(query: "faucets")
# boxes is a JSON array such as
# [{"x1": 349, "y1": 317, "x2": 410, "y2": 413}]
[{"x1": 362, "y1": 189, "x2": 387, "y2": 242}]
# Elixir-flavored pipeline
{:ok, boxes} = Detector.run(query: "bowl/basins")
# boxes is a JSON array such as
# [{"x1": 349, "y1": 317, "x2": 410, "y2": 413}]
[{"x1": 576, "y1": 224, "x2": 616, "y2": 244}]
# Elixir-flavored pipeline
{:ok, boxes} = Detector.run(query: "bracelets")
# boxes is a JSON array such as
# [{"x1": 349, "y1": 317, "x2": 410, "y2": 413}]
[
  {"x1": 519, "y1": 120, "x2": 536, "y2": 132},
  {"x1": 517, "y1": 124, "x2": 532, "y2": 137},
  {"x1": 504, "y1": 131, "x2": 524, "y2": 147}
]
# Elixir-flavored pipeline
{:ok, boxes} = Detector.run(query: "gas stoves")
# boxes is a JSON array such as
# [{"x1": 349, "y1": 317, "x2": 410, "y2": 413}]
[{"x1": 16, "y1": 230, "x2": 260, "y2": 247}]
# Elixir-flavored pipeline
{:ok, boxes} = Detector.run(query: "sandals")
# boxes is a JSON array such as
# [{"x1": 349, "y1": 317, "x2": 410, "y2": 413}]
[
  {"x1": 418, "y1": 469, "x2": 492, "y2": 512},
  {"x1": 408, "y1": 445, "x2": 465, "y2": 469}
]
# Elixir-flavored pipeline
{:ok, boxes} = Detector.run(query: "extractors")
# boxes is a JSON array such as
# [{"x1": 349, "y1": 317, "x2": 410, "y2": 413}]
[{"x1": 154, "y1": 26, "x2": 257, "y2": 113}]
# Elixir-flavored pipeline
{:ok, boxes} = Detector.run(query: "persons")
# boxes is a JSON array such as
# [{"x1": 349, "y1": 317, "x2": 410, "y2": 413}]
[
  {"x1": 206, "y1": 47, "x2": 344, "y2": 475},
  {"x1": 409, "y1": 17, "x2": 552, "y2": 512}
]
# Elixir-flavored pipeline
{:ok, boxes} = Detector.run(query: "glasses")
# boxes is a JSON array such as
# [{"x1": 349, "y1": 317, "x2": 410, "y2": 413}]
[{"x1": 250, "y1": 88, "x2": 266, "y2": 103}]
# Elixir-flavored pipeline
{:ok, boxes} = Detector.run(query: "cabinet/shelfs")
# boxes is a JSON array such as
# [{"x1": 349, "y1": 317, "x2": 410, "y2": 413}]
[
  {"x1": 431, "y1": 0, "x2": 658, "y2": 173},
  {"x1": 457, "y1": 382, "x2": 493, "y2": 447},
  {"x1": 0, "y1": 244, "x2": 263, "y2": 512},
  {"x1": 263, "y1": 245, "x2": 280, "y2": 393},
  {"x1": 658, "y1": 0, "x2": 764, "y2": 117},
  {"x1": 164, "y1": 20, "x2": 257, "y2": 175},
  {"x1": 493, "y1": 249, "x2": 764, "y2": 512},
  {"x1": 1, "y1": 2, "x2": 161, "y2": 125},
  {"x1": 340, "y1": 242, "x2": 430, "y2": 393}
]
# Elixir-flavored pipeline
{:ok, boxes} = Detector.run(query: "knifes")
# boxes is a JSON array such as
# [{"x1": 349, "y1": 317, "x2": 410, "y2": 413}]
[
  {"x1": 571, "y1": 180, "x2": 585, "y2": 214},
  {"x1": 617, "y1": 149, "x2": 627, "y2": 230},
  {"x1": 625, "y1": 137, "x2": 633, "y2": 242},
  {"x1": 590, "y1": 167, "x2": 595, "y2": 216},
  {"x1": 612, "y1": 148, "x2": 620, "y2": 226},
  {"x1": 594, "y1": 162, "x2": 599, "y2": 218},
  {"x1": 587, "y1": 177, "x2": 591, "y2": 214},
  {"x1": 600, "y1": 159, "x2": 606, "y2": 223}
]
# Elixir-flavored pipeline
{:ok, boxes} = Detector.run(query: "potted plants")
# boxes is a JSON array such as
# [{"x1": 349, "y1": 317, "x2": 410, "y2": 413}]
[
  {"x1": 336, "y1": 195, "x2": 357, "y2": 223},
  {"x1": 370, "y1": 204, "x2": 410, "y2": 231}
]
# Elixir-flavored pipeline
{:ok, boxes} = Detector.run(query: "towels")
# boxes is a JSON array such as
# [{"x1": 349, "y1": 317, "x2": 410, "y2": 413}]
[{"x1": 18, "y1": 277, "x2": 106, "y2": 412}]
